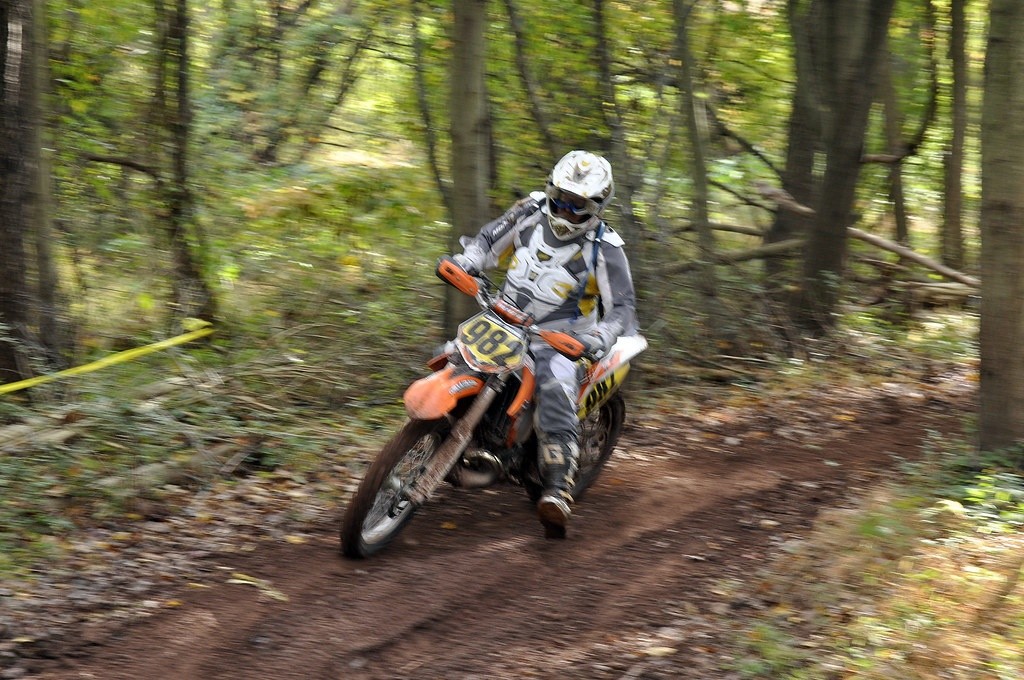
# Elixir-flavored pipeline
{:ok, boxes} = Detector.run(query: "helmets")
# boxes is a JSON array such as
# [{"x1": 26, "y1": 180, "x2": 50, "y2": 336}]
[{"x1": 544, "y1": 150, "x2": 614, "y2": 242}]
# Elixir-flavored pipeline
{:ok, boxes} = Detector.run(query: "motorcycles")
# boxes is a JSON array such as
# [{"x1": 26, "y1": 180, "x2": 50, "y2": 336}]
[{"x1": 337, "y1": 254, "x2": 648, "y2": 558}]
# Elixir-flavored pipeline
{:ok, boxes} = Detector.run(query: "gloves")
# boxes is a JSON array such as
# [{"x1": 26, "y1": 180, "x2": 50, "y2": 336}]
[
  {"x1": 452, "y1": 235, "x2": 490, "y2": 272},
  {"x1": 574, "y1": 320, "x2": 625, "y2": 359}
]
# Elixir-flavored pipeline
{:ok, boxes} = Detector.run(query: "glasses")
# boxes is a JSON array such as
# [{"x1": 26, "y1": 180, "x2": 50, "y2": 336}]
[{"x1": 548, "y1": 196, "x2": 592, "y2": 223}]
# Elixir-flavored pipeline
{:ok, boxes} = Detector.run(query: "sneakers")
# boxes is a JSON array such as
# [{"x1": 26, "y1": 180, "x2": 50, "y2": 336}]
[{"x1": 534, "y1": 468, "x2": 576, "y2": 545}]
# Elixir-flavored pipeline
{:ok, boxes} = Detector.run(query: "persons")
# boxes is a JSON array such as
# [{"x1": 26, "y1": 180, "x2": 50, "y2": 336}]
[{"x1": 437, "y1": 150, "x2": 636, "y2": 538}]
